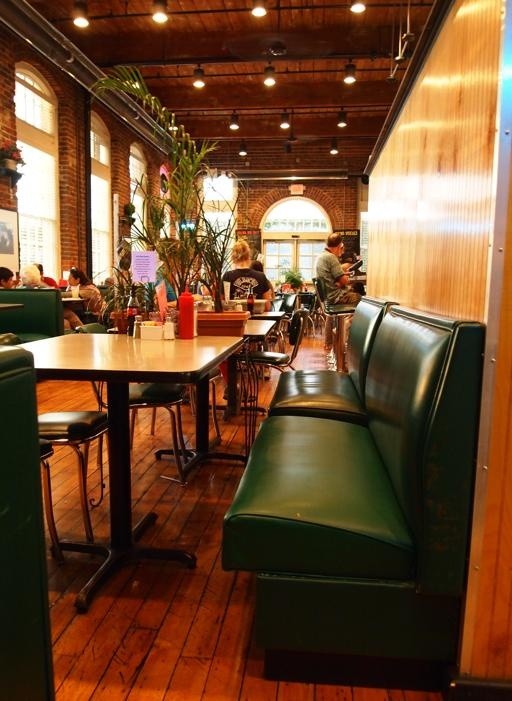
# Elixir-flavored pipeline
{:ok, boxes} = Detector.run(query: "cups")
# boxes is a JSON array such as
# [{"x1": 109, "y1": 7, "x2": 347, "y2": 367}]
[{"x1": 71, "y1": 286, "x2": 80, "y2": 298}]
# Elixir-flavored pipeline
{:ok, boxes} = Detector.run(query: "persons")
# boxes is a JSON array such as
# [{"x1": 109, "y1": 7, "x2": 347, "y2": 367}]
[
  {"x1": 220, "y1": 239, "x2": 272, "y2": 301},
  {"x1": 34, "y1": 264, "x2": 60, "y2": 289},
  {"x1": 0, "y1": 267, "x2": 14, "y2": 289},
  {"x1": 323, "y1": 237, "x2": 349, "y2": 351},
  {"x1": 66, "y1": 267, "x2": 105, "y2": 324},
  {"x1": 249, "y1": 261, "x2": 275, "y2": 299},
  {"x1": 15, "y1": 264, "x2": 83, "y2": 330},
  {"x1": 316, "y1": 231, "x2": 364, "y2": 345}
]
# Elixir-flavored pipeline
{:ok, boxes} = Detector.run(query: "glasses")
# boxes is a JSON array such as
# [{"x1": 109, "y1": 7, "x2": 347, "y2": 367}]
[{"x1": 72, "y1": 265, "x2": 77, "y2": 272}]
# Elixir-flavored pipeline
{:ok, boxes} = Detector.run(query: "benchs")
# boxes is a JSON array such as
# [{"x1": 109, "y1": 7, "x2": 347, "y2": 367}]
[
  {"x1": 218, "y1": 297, "x2": 486, "y2": 678},
  {"x1": 265, "y1": 295, "x2": 397, "y2": 419}
]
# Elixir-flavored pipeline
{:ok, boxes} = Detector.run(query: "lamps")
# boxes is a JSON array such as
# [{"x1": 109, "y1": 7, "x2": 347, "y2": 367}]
[
  {"x1": 230, "y1": 107, "x2": 348, "y2": 131},
  {"x1": 71, "y1": 0, "x2": 368, "y2": 27},
  {"x1": 239, "y1": 138, "x2": 339, "y2": 157},
  {"x1": 192, "y1": 57, "x2": 357, "y2": 90}
]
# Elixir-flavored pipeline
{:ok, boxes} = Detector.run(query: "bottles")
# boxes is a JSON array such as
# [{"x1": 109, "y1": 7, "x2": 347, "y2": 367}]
[
  {"x1": 179, "y1": 283, "x2": 194, "y2": 339},
  {"x1": 246, "y1": 284, "x2": 255, "y2": 317},
  {"x1": 128, "y1": 290, "x2": 141, "y2": 335},
  {"x1": 193, "y1": 302, "x2": 198, "y2": 337},
  {"x1": 133, "y1": 315, "x2": 142, "y2": 338},
  {"x1": 164, "y1": 316, "x2": 175, "y2": 341}
]
